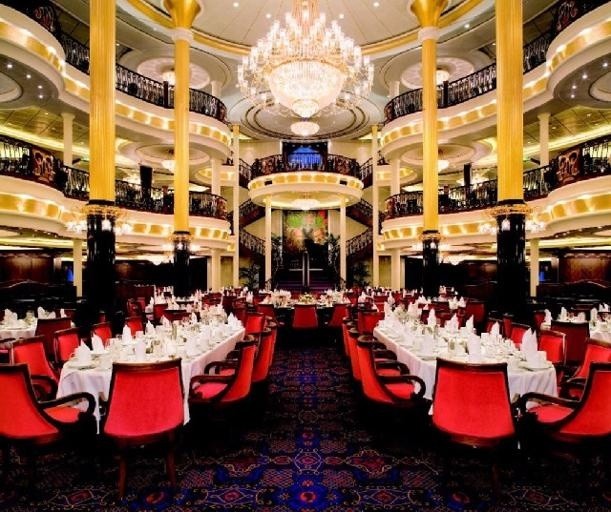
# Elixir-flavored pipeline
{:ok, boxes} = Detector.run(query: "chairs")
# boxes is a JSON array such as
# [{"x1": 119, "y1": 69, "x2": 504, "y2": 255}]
[
  {"x1": 1, "y1": 284, "x2": 279, "y2": 502},
  {"x1": 189, "y1": 281, "x2": 423, "y2": 330},
  {"x1": 341, "y1": 284, "x2": 611, "y2": 502}
]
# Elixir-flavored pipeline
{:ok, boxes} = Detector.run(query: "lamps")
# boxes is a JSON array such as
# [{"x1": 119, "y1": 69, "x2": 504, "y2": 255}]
[{"x1": 236, "y1": 0, "x2": 375, "y2": 119}]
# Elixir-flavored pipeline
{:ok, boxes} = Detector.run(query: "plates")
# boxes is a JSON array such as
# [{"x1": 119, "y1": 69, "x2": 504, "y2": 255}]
[{"x1": 6, "y1": 327, "x2": 27, "y2": 329}]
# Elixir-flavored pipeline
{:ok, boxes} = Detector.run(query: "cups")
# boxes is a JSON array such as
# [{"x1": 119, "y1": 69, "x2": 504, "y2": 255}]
[
  {"x1": 596, "y1": 313, "x2": 611, "y2": 332},
  {"x1": 153, "y1": 285, "x2": 205, "y2": 311},
  {"x1": 71, "y1": 310, "x2": 244, "y2": 371},
  {"x1": 563, "y1": 312, "x2": 575, "y2": 322},
  {"x1": 401, "y1": 317, "x2": 547, "y2": 368},
  {"x1": 26, "y1": 309, "x2": 35, "y2": 327}
]
